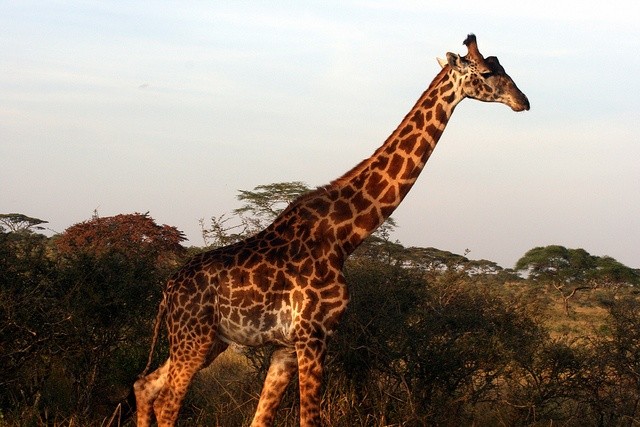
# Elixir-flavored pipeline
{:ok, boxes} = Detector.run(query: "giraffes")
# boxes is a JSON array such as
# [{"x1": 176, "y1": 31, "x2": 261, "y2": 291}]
[{"x1": 133, "y1": 32, "x2": 532, "y2": 426}]
